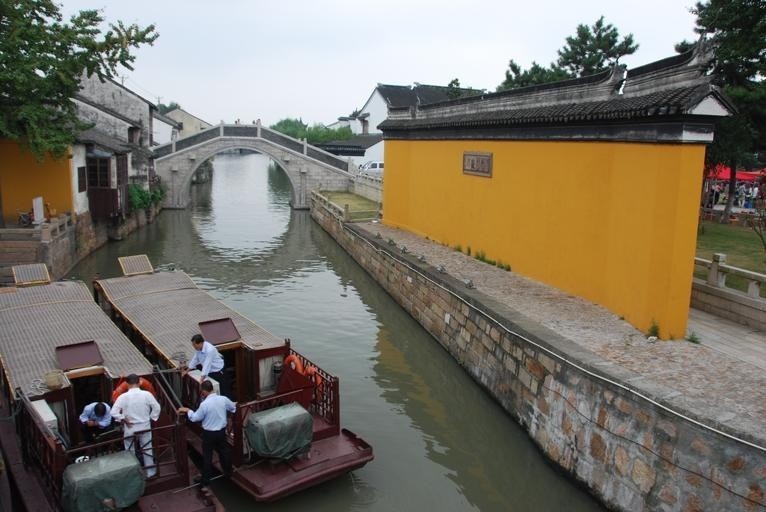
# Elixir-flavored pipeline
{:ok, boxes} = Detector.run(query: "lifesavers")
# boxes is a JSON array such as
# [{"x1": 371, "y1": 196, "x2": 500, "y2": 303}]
[
  {"x1": 285, "y1": 355, "x2": 303, "y2": 374},
  {"x1": 111, "y1": 376, "x2": 157, "y2": 405},
  {"x1": 304, "y1": 366, "x2": 323, "y2": 403}
]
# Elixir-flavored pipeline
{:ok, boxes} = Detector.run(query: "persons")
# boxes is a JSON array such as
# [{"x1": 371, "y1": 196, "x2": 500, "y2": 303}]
[
  {"x1": 702, "y1": 176, "x2": 766, "y2": 210},
  {"x1": 78, "y1": 402, "x2": 112, "y2": 442},
  {"x1": 110, "y1": 373, "x2": 162, "y2": 480},
  {"x1": 181, "y1": 334, "x2": 227, "y2": 396},
  {"x1": 176, "y1": 380, "x2": 238, "y2": 487}
]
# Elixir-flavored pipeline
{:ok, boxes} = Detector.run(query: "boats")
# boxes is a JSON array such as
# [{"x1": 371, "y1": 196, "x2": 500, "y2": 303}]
[
  {"x1": 0, "y1": 263, "x2": 226, "y2": 511},
  {"x1": 92, "y1": 252, "x2": 377, "y2": 503}
]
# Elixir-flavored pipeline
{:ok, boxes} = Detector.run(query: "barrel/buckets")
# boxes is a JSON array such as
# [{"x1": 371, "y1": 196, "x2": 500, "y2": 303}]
[{"x1": 45, "y1": 370, "x2": 64, "y2": 390}]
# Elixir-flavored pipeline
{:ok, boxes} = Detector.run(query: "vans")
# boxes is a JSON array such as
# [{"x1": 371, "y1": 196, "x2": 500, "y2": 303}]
[{"x1": 359, "y1": 161, "x2": 384, "y2": 178}]
